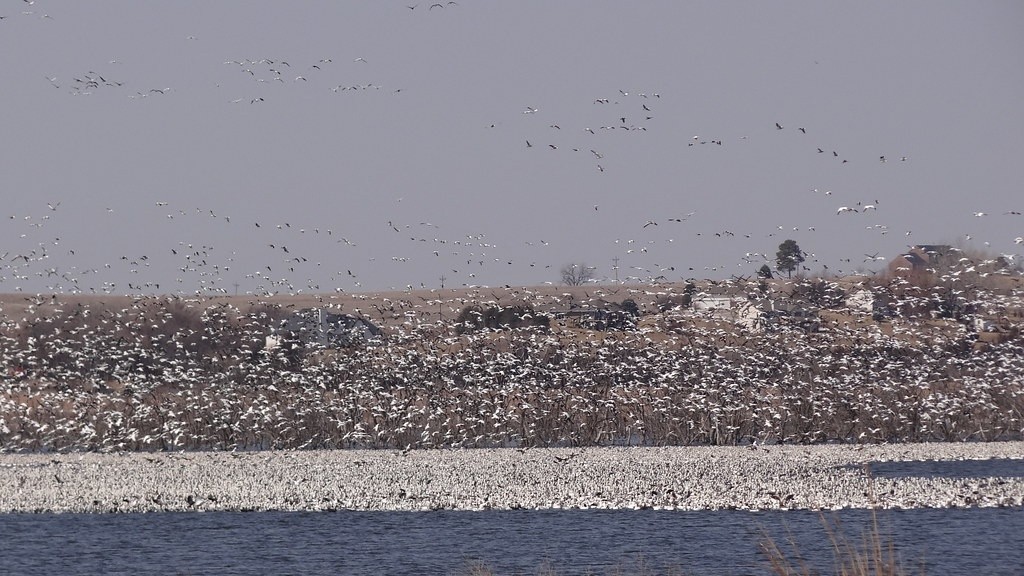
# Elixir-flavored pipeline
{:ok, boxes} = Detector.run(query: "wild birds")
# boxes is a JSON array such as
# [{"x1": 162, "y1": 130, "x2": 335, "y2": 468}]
[{"x1": 2, "y1": 1, "x2": 1024, "y2": 514}]
